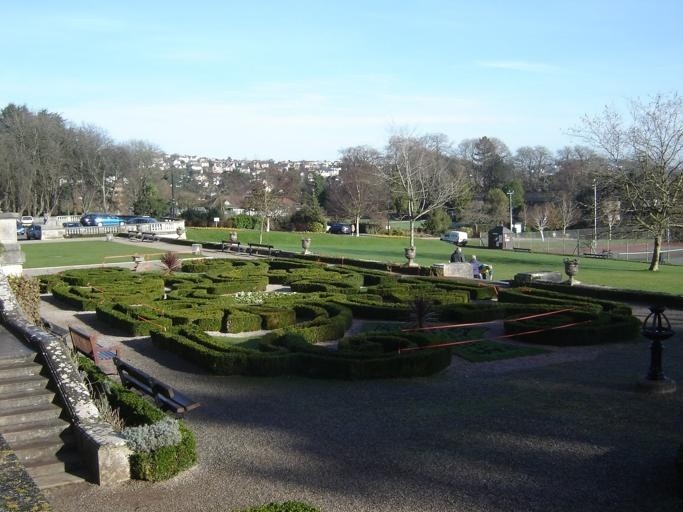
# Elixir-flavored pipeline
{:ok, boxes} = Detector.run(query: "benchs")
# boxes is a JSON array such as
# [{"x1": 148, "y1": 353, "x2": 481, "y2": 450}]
[
  {"x1": 128, "y1": 230, "x2": 159, "y2": 243},
  {"x1": 318, "y1": 254, "x2": 420, "y2": 276},
  {"x1": 221, "y1": 240, "x2": 274, "y2": 257},
  {"x1": 41, "y1": 317, "x2": 202, "y2": 423}
]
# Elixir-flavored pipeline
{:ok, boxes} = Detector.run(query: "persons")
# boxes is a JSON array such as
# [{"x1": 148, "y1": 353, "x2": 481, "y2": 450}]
[
  {"x1": 469, "y1": 255, "x2": 479, "y2": 279},
  {"x1": 478, "y1": 263, "x2": 493, "y2": 280},
  {"x1": 450, "y1": 246, "x2": 464, "y2": 263}
]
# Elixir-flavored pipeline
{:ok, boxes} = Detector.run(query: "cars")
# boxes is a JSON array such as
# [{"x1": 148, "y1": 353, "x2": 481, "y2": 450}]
[
  {"x1": 63, "y1": 213, "x2": 158, "y2": 228},
  {"x1": 17, "y1": 216, "x2": 41, "y2": 239},
  {"x1": 330, "y1": 223, "x2": 352, "y2": 234}
]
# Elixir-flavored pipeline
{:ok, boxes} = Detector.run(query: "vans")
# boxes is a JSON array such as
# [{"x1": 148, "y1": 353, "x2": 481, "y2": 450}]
[{"x1": 440, "y1": 230, "x2": 467, "y2": 246}]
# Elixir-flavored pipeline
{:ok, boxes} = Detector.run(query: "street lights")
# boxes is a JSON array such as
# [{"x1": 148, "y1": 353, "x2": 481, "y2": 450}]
[{"x1": 164, "y1": 171, "x2": 176, "y2": 218}]
